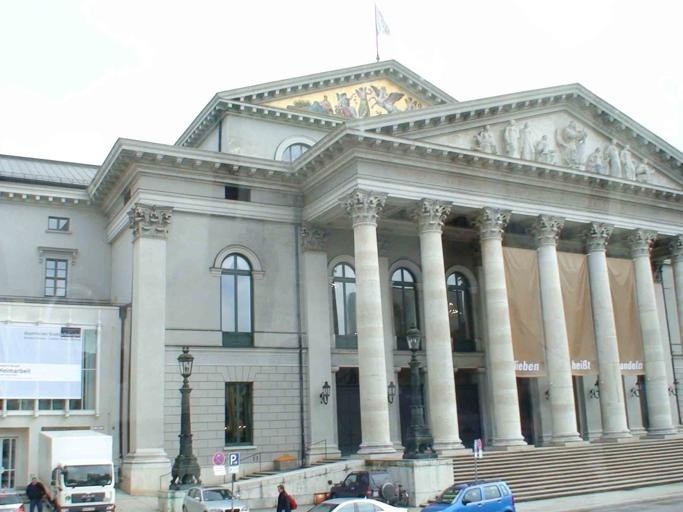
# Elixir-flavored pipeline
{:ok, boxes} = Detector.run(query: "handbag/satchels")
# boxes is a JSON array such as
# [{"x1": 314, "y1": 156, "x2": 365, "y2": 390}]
[{"x1": 288, "y1": 495, "x2": 297, "y2": 509}]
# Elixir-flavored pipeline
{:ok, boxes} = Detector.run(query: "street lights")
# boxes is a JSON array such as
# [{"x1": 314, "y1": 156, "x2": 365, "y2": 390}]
[
  {"x1": 170, "y1": 345, "x2": 201, "y2": 490},
  {"x1": 403, "y1": 322, "x2": 438, "y2": 459}
]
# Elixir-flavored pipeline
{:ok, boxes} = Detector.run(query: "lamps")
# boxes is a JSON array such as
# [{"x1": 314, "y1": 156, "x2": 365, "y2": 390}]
[
  {"x1": 388, "y1": 382, "x2": 396, "y2": 403},
  {"x1": 320, "y1": 381, "x2": 331, "y2": 405},
  {"x1": 590, "y1": 380, "x2": 600, "y2": 399},
  {"x1": 631, "y1": 380, "x2": 641, "y2": 398},
  {"x1": 667, "y1": 378, "x2": 680, "y2": 397}
]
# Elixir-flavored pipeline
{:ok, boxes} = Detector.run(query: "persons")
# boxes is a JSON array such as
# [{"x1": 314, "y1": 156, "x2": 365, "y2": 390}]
[
  {"x1": 310, "y1": 85, "x2": 423, "y2": 122},
  {"x1": 275, "y1": 484, "x2": 291, "y2": 512},
  {"x1": 474, "y1": 118, "x2": 655, "y2": 183},
  {"x1": 25, "y1": 477, "x2": 45, "y2": 511}
]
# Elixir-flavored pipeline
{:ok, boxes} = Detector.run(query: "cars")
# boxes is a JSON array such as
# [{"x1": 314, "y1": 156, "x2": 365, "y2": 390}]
[
  {"x1": 183, "y1": 486, "x2": 250, "y2": 512},
  {"x1": 0, "y1": 489, "x2": 24, "y2": 512},
  {"x1": 421, "y1": 479, "x2": 515, "y2": 512},
  {"x1": 307, "y1": 497, "x2": 408, "y2": 512}
]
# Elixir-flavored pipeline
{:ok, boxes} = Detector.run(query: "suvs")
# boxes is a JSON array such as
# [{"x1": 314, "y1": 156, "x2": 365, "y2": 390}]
[{"x1": 329, "y1": 468, "x2": 397, "y2": 504}]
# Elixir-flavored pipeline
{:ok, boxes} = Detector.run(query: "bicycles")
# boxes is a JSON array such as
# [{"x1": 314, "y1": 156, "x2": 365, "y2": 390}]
[{"x1": 398, "y1": 484, "x2": 409, "y2": 505}]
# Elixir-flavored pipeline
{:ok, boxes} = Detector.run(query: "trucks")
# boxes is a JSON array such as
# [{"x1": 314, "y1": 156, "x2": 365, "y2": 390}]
[{"x1": 38, "y1": 429, "x2": 116, "y2": 512}]
